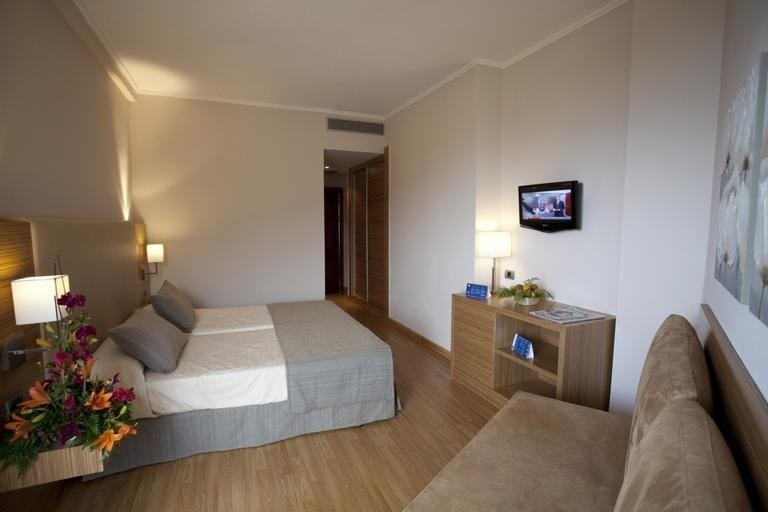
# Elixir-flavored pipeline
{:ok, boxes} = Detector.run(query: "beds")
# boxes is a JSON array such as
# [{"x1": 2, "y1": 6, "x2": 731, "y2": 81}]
[{"x1": 81, "y1": 300, "x2": 400, "y2": 483}]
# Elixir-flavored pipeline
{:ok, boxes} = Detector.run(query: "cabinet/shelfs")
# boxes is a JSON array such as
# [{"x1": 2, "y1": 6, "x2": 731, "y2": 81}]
[{"x1": 450, "y1": 294, "x2": 616, "y2": 412}]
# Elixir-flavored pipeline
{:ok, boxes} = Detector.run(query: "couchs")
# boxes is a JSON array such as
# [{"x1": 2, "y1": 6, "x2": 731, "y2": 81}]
[{"x1": 401, "y1": 305, "x2": 768, "y2": 512}]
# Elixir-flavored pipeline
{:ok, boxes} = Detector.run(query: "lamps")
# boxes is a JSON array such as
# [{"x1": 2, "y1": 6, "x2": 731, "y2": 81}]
[
  {"x1": 0, "y1": 275, "x2": 73, "y2": 370},
  {"x1": 139, "y1": 244, "x2": 164, "y2": 279},
  {"x1": 477, "y1": 231, "x2": 511, "y2": 295}
]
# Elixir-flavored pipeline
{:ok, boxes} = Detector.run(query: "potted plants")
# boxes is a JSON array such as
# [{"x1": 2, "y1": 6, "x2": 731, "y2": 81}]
[{"x1": 494, "y1": 286, "x2": 513, "y2": 303}]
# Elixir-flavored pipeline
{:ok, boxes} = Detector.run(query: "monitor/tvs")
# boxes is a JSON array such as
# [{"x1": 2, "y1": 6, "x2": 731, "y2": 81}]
[{"x1": 518, "y1": 180, "x2": 580, "y2": 233}]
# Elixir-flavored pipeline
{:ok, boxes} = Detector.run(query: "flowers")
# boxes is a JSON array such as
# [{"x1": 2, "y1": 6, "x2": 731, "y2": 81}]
[
  {"x1": 511, "y1": 278, "x2": 553, "y2": 303},
  {"x1": 0, "y1": 293, "x2": 139, "y2": 479}
]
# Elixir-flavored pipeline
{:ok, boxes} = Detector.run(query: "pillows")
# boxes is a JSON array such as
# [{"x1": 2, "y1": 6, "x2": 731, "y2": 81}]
[
  {"x1": 613, "y1": 400, "x2": 751, "y2": 512},
  {"x1": 149, "y1": 280, "x2": 196, "y2": 333},
  {"x1": 623, "y1": 314, "x2": 712, "y2": 478},
  {"x1": 107, "y1": 308, "x2": 188, "y2": 373}
]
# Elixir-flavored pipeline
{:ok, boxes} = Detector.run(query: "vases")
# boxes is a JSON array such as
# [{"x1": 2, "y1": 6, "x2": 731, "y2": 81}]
[{"x1": 519, "y1": 297, "x2": 540, "y2": 305}]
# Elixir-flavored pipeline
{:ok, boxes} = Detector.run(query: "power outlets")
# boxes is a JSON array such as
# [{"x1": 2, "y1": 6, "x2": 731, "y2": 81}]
[{"x1": 504, "y1": 270, "x2": 514, "y2": 280}]
